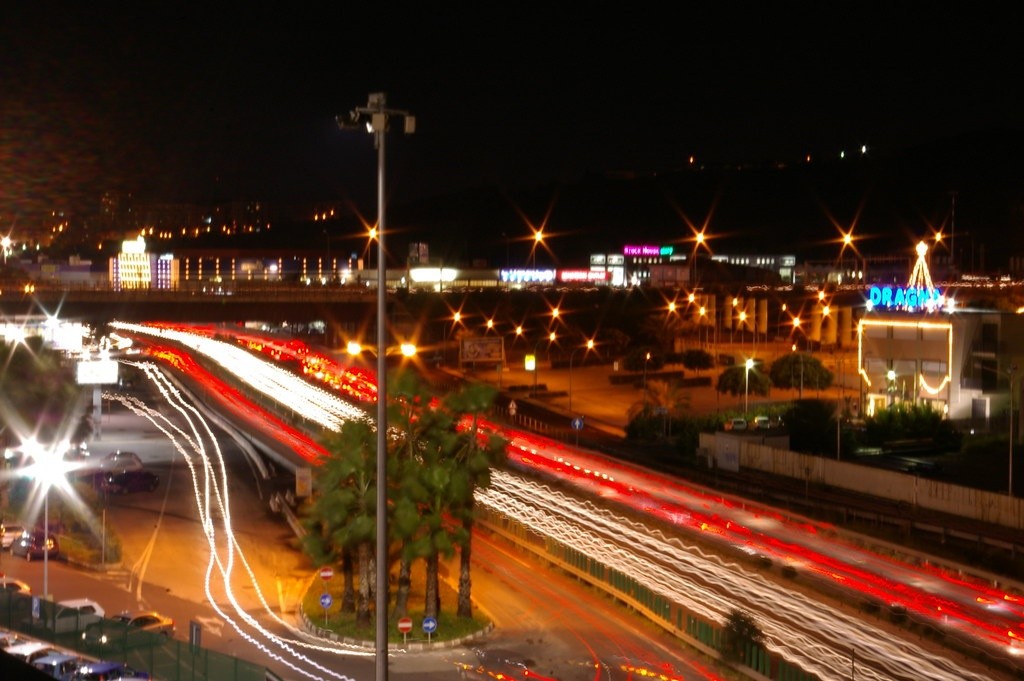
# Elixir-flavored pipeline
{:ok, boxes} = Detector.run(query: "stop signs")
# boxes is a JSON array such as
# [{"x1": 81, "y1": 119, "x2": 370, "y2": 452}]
[
  {"x1": 398, "y1": 617, "x2": 413, "y2": 633},
  {"x1": 320, "y1": 567, "x2": 334, "y2": 580}
]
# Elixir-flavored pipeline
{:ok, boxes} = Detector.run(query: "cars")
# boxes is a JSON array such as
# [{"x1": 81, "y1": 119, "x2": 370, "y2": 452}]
[
  {"x1": 0, "y1": 523, "x2": 25, "y2": 548},
  {"x1": 0, "y1": 626, "x2": 150, "y2": 681},
  {"x1": 22, "y1": 597, "x2": 104, "y2": 638},
  {"x1": 9, "y1": 529, "x2": 59, "y2": 563},
  {"x1": 102, "y1": 469, "x2": 161, "y2": 496},
  {"x1": 0, "y1": 576, "x2": 31, "y2": 609},
  {"x1": 100, "y1": 451, "x2": 143, "y2": 473},
  {"x1": 80, "y1": 610, "x2": 176, "y2": 654}
]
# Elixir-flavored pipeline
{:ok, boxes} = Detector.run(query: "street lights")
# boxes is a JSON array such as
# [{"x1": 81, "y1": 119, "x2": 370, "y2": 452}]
[
  {"x1": 443, "y1": 314, "x2": 462, "y2": 367},
  {"x1": 568, "y1": 340, "x2": 594, "y2": 422},
  {"x1": 642, "y1": 351, "x2": 652, "y2": 418},
  {"x1": 788, "y1": 344, "x2": 798, "y2": 398},
  {"x1": 973, "y1": 361, "x2": 1024, "y2": 496},
  {"x1": 743, "y1": 358, "x2": 755, "y2": 415},
  {"x1": 335, "y1": 91, "x2": 416, "y2": 681},
  {"x1": 29, "y1": 449, "x2": 63, "y2": 600},
  {"x1": 533, "y1": 331, "x2": 555, "y2": 407}
]
